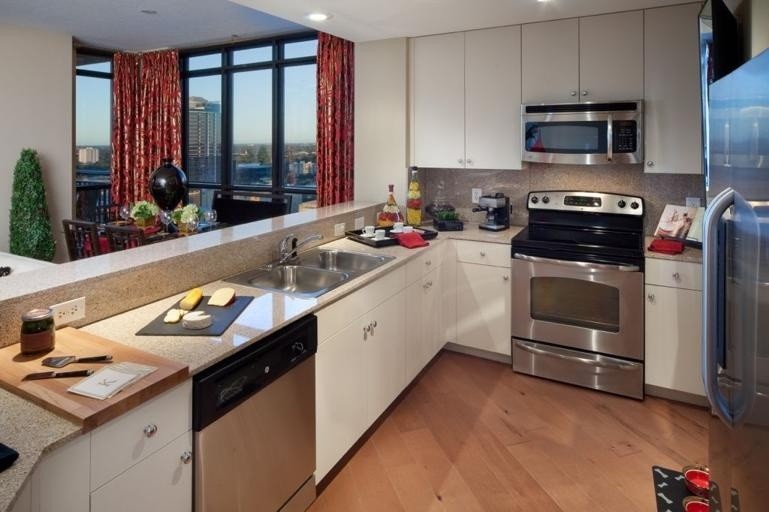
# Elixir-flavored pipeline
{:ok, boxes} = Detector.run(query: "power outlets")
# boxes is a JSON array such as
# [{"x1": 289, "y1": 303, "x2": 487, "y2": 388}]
[
  {"x1": 49, "y1": 296, "x2": 86, "y2": 326},
  {"x1": 686, "y1": 198, "x2": 701, "y2": 207},
  {"x1": 472, "y1": 188, "x2": 481, "y2": 203}
]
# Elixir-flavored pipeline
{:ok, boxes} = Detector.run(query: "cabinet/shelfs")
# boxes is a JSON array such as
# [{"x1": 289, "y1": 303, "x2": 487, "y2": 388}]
[
  {"x1": 406, "y1": 238, "x2": 456, "y2": 387},
  {"x1": 644, "y1": 1, "x2": 704, "y2": 174},
  {"x1": 408, "y1": 25, "x2": 527, "y2": 170},
  {"x1": 456, "y1": 240, "x2": 511, "y2": 363},
  {"x1": 13, "y1": 377, "x2": 192, "y2": 512},
  {"x1": 644, "y1": 257, "x2": 710, "y2": 408},
  {"x1": 522, "y1": 8, "x2": 644, "y2": 105},
  {"x1": 315, "y1": 264, "x2": 406, "y2": 489}
]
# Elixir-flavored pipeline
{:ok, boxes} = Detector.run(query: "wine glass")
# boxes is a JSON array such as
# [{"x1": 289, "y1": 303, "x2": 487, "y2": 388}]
[{"x1": 119, "y1": 203, "x2": 218, "y2": 237}]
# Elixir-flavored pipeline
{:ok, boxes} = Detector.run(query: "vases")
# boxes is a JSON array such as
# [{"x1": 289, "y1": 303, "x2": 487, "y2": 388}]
[
  {"x1": 178, "y1": 221, "x2": 192, "y2": 236},
  {"x1": 137, "y1": 218, "x2": 155, "y2": 229}
]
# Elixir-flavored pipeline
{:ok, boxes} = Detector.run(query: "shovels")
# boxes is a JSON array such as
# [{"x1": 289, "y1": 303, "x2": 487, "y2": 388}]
[
  {"x1": 42, "y1": 355, "x2": 112, "y2": 367},
  {"x1": 21, "y1": 369, "x2": 95, "y2": 383}
]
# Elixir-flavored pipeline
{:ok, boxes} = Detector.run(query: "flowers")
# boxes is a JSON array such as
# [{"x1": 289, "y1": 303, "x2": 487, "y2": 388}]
[
  {"x1": 131, "y1": 201, "x2": 159, "y2": 220},
  {"x1": 172, "y1": 204, "x2": 204, "y2": 224}
]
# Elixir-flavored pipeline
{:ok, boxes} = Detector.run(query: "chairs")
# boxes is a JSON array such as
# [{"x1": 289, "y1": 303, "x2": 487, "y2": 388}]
[
  {"x1": 62, "y1": 220, "x2": 99, "y2": 262},
  {"x1": 105, "y1": 225, "x2": 144, "y2": 252}
]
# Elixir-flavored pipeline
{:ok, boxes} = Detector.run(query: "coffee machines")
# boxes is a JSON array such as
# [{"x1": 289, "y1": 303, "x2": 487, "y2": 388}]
[{"x1": 470, "y1": 192, "x2": 513, "y2": 232}]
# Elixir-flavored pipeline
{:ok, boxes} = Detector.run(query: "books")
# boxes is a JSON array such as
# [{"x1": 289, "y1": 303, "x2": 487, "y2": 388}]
[{"x1": 654, "y1": 203, "x2": 705, "y2": 244}]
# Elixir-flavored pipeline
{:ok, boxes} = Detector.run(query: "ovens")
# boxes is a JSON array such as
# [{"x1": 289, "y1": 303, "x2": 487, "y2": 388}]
[{"x1": 510, "y1": 254, "x2": 645, "y2": 401}]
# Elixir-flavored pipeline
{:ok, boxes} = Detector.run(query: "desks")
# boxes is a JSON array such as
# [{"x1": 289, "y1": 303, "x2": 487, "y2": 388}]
[{"x1": 83, "y1": 221, "x2": 161, "y2": 257}]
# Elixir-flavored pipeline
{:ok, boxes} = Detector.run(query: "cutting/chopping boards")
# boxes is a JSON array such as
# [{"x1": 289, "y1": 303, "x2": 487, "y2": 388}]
[
  {"x1": 135, "y1": 295, "x2": 253, "y2": 338},
  {"x1": 0, "y1": 327, "x2": 189, "y2": 429}
]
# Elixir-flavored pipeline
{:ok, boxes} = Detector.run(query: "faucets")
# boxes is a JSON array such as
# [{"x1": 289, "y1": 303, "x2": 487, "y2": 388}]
[{"x1": 278, "y1": 231, "x2": 325, "y2": 264}]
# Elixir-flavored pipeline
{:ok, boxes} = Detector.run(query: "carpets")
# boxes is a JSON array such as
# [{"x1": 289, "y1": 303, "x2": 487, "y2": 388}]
[{"x1": 652, "y1": 465, "x2": 695, "y2": 512}]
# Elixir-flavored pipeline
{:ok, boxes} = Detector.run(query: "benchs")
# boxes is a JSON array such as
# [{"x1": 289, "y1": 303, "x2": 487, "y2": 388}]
[{"x1": 212, "y1": 191, "x2": 292, "y2": 225}]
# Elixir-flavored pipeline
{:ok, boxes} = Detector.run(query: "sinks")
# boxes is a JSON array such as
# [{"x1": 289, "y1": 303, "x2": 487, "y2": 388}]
[
  {"x1": 300, "y1": 250, "x2": 397, "y2": 273},
  {"x1": 247, "y1": 265, "x2": 364, "y2": 301}
]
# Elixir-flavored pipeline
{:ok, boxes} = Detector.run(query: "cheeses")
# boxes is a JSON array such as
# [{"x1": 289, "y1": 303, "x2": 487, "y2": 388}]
[{"x1": 163, "y1": 288, "x2": 236, "y2": 329}]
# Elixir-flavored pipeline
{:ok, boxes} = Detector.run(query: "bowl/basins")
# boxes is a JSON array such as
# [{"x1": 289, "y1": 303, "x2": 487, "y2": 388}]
[
  {"x1": 683, "y1": 495, "x2": 709, "y2": 512},
  {"x1": 682, "y1": 463, "x2": 710, "y2": 498}
]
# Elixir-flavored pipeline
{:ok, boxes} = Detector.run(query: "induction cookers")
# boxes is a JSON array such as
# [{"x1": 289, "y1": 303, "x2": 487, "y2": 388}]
[{"x1": 510, "y1": 223, "x2": 646, "y2": 257}]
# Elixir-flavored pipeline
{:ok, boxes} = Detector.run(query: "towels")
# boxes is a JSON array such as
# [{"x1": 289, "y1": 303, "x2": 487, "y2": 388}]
[
  {"x1": 400, "y1": 233, "x2": 429, "y2": 248},
  {"x1": 648, "y1": 239, "x2": 683, "y2": 254}
]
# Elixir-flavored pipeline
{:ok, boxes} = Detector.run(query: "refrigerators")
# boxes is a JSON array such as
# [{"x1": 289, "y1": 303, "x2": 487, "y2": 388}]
[{"x1": 700, "y1": 45, "x2": 768, "y2": 511}]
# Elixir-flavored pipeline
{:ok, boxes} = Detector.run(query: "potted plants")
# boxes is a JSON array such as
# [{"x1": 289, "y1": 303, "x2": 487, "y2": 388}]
[{"x1": 433, "y1": 211, "x2": 463, "y2": 231}]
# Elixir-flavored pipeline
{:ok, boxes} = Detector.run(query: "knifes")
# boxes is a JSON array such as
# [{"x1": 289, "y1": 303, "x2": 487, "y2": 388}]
[{"x1": 20, "y1": 368, "x2": 94, "y2": 383}]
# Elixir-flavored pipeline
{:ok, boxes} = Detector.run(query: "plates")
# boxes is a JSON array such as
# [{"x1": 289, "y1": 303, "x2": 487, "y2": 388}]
[
  {"x1": 371, "y1": 236, "x2": 390, "y2": 241},
  {"x1": 360, "y1": 233, "x2": 376, "y2": 238},
  {"x1": 389, "y1": 229, "x2": 402, "y2": 234}
]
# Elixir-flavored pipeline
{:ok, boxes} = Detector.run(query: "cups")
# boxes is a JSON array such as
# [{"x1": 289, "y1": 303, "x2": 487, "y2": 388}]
[
  {"x1": 403, "y1": 226, "x2": 414, "y2": 233},
  {"x1": 362, "y1": 225, "x2": 375, "y2": 235},
  {"x1": 392, "y1": 222, "x2": 403, "y2": 231},
  {"x1": 374, "y1": 230, "x2": 386, "y2": 239}
]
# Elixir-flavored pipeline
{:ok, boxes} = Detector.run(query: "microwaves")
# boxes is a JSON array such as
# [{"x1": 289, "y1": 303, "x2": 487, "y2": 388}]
[{"x1": 520, "y1": 98, "x2": 645, "y2": 167}]
